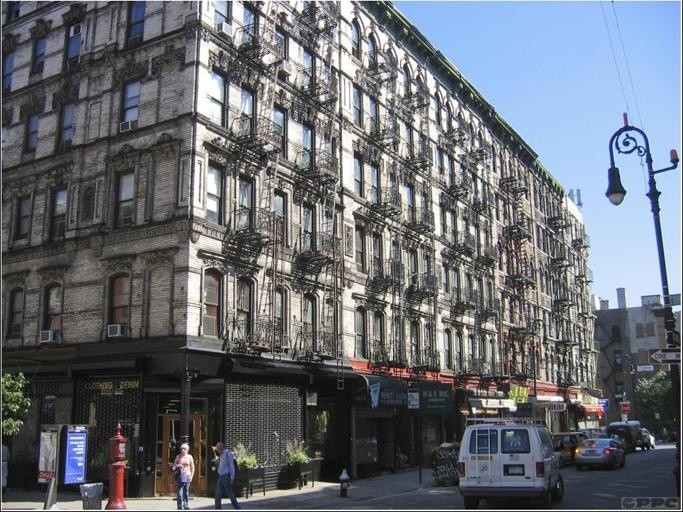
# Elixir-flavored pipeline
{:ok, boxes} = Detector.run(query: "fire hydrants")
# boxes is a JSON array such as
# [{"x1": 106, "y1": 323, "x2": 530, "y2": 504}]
[
  {"x1": 103, "y1": 423, "x2": 128, "y2": 509},
  {"x1": 339, "y1": 469, "x2": 350, "y2": 496}
]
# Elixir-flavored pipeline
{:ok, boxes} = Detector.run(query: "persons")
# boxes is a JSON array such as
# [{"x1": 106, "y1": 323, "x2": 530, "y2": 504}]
[
  {"x1": 212, "y1": 439, "x2": 241, "y2": 509},
  {"x1": 171, "y1": 441, "x2": 195, "y2": 509}
]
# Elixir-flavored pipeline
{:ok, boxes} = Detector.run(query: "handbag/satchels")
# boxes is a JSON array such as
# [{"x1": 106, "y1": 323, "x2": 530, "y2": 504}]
[{"x1": 174, "y1": 467, "x2": 181, "y2": 480}]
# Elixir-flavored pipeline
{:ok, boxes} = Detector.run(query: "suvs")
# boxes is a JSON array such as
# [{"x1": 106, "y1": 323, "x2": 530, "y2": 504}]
[
  {"x1": 556, "y1": 431, "x2": 587, "y2": 469},
  {"x1": 458, "y1": 417, "x2": 564, "y2": 508}
]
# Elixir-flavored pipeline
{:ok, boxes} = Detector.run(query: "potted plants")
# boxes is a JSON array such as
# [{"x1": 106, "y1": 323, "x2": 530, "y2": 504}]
[
  {"x1": 210, "y1": 439, "x2": 266, "y2": 500},
  {"x1": 284, "y1": 438, "x2": 314, "y2": 489}
]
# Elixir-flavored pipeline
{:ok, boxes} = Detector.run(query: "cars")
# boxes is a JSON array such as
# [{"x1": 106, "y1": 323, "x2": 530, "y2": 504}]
[
  {"x1": 574, "y1": 438, "x2": 624, "y2": 471},
  {"x1": 587, "y1": 417, "x2": 655, "y2": 451}
]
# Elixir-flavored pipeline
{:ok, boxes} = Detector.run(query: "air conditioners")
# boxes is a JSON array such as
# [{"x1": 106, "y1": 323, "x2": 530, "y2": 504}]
[
  {"x1": 106, "y1": 323, "x2": 128, "y2": 337},
  {"x1": 119, "y1": 120, "x2": 137, "y2": 134},
  {"x1": 39, "y1": 329, "x2": 60, "y2": 344},
  {"x1": 279, "y1": 59, "x2": 293, "y2": 77}
]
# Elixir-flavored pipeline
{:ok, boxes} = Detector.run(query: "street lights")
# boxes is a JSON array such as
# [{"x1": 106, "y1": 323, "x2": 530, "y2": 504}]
[{"x1": 605, "y1": 112, "x2": 681, "y2": 497}]
